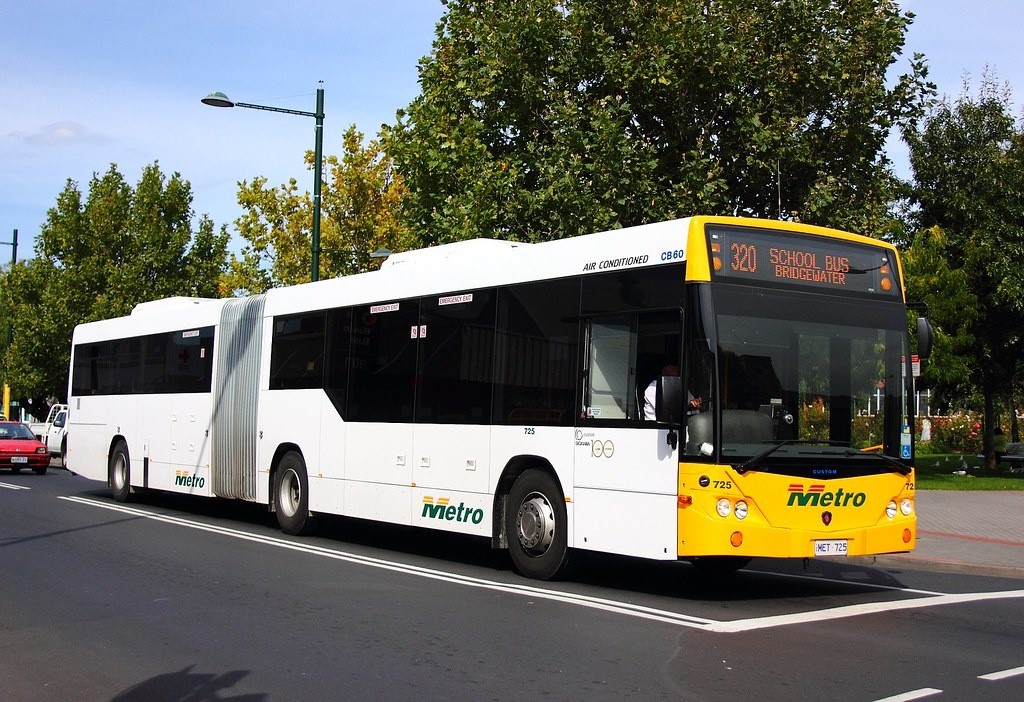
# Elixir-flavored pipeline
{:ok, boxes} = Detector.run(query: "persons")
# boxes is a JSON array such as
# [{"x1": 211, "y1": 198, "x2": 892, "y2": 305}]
[{"x1": 644, "y1": 365, "x2": 702, "y2": 421}]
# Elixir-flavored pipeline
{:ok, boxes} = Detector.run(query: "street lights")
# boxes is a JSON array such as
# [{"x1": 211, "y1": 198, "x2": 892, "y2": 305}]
[{"x1": 202, "y1": 79, "x2": 325, "y2": 282}]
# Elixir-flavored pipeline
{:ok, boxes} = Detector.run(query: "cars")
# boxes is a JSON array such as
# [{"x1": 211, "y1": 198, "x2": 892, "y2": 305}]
[{"x1": 0, "y1": 420, "x2": 50, "y2": 475}]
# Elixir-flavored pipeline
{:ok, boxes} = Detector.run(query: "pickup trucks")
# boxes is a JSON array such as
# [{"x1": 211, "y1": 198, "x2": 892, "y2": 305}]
[{"x1": 28, "y1": 404, "x2": 67, "y2": 468}]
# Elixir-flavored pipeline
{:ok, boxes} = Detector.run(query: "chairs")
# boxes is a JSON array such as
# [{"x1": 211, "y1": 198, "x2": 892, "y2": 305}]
[{"x1": 635, "y1": 380, "x2": 647, "y2": 421}]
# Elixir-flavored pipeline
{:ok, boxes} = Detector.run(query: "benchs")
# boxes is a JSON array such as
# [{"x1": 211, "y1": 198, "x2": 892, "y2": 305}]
[{"x1": 1000, "y1": 442, "x2": 1024, "y2": 469}]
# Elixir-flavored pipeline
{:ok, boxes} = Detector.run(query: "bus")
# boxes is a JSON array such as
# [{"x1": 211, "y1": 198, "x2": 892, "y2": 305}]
[{"x1": 66, "y1": 216, "x2": 934, "y2": 578}]
